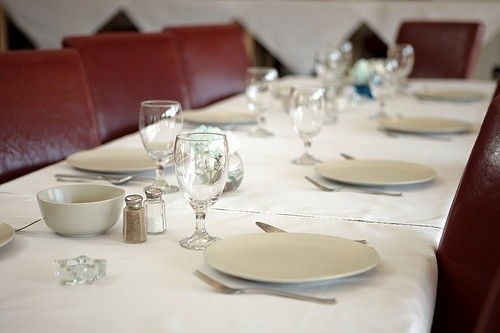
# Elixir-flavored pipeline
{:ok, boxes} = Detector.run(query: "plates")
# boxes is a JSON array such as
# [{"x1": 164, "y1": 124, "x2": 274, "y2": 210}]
[
  {"x1": 376, "y1": 117, "x2": 475, "y2": 133},
  {"x1": 0, "y1": 221, "x2": 15, "y2": 248},
  {"x1": 315, "y1": 159, "x2": 437, "y2": 188},
  {"x1": 412, "y1": 88, "x2": 488, "y2": 99},
  {"x1": 68, "y1": 150, "x2": 174, "y2": 170},
  {"x1": 183, "y1": 112, "x2": 256, "y2": 124},
  {"x1": 202, "y1": 233, "x2": 380, "y2": 285}
]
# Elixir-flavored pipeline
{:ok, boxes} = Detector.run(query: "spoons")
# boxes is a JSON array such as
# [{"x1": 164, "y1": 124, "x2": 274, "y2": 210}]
[
  {"x1": 254, "y1": 221, "x2": 367, "y2": 244},
  {"x1": 57, "y1": 176, "x2": 138, "y2": 185}
]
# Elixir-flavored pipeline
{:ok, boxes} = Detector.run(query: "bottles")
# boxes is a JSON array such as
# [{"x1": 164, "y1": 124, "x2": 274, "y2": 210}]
[
  {"x1": 145, "y1": 187, "x2": 167, "y2": 235},
  {"x1": 123, "y1": 195, "x2": 149, "y2": 244}
]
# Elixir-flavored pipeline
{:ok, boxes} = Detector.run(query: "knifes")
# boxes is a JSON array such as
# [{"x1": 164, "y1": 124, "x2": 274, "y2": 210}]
[{"x1": 54, "y1": 173, "x2": 156, "y2": 183}]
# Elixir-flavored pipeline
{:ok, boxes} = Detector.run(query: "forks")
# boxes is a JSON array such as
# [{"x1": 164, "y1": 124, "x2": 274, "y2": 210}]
[
  {"x1": 193, "y1": 271, "x2": 336, "y2": 304},
  {"x1": 304, "y1": 177, "x2": 402, "y2": 198}
]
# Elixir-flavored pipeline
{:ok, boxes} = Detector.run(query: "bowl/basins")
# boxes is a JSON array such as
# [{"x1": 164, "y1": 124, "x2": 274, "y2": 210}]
[{"x1": 36, "y1": 184, "x2": 126, "y2": 238}]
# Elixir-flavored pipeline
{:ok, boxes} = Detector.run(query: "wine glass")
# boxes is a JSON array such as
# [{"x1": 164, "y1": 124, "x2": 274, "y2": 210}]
[
  {"x1": 246, "y1": 67, "x2": 279, "y2": 136},
  {"x1": 174, "y1": 132, "x2": 230, "y2": 250},
  {"x1": 290, "y1": 85, "x2": 325, "y2": 165},
  {"x1": 140, "y1": 99, "x2": 181, "y2": 192},
  {"x1": 314, "y1": 36, "x2": 417, "y2": 111}
]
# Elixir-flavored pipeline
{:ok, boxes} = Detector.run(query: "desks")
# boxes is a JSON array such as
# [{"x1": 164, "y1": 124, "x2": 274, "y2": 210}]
[
  {"x1": 0, "y1": 77, "x2": 497, "y2": 255},
  {"x1": 0, "y1": 189, "x2": 438, "y2": 333}
]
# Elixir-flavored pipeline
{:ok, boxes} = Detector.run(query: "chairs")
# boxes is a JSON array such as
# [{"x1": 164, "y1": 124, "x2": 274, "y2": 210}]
[{"x1": 0, "y1": 21, "x2": 500, "y2": 333}]
